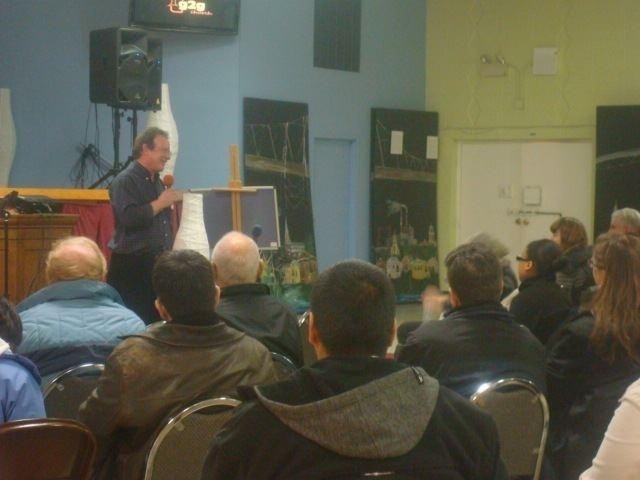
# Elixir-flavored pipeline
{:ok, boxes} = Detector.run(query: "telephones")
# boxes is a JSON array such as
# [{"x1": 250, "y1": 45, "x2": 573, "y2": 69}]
[{"x1": 12, "y1": 195, "x2": 63, "y2": 214}]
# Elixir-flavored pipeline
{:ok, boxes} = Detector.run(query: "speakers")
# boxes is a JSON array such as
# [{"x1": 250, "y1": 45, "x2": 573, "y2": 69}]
[
  {"x1": 136, "y1": 37, "x2": 163, "y2": 111},
  {"x1": 89, "y1": 27, "x2": 148, "y2": 110}
]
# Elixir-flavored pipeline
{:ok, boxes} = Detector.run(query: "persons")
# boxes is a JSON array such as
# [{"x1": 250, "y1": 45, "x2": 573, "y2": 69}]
[
  {"x1": 394, "y1": 209, "x2": 639, "y2": 479},
  {"x1": 200, "y1": 256, "x2": 511, "y2": 478},
  {"x1": 1, "y1": 230, "x2": 302, "y2": 479},
  {"x1": 107, "y1": 125, "x2": 183, "y2": 324}
]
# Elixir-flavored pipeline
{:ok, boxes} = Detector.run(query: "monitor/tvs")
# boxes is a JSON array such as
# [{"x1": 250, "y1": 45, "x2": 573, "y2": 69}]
[{"x1": 128, "y1": 0, "x2": 240, "y2": 35}]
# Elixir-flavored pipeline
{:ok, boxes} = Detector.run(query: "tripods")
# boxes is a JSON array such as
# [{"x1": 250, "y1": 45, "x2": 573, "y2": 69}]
[
  {"x1": 88, "y1": 106, "x2": 122, "y2": 189},
  {"x1": 118, "y1": 111, "x2": 138, "y2": 177}
]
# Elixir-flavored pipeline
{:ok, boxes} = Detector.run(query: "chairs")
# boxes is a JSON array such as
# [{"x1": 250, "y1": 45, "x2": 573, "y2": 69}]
[
  {"x1": 142, "y1": 395, "x2": 241, "y2": 478},
  {"x1": 44, "y1": 364, "x2": 105, "y2": 421},
  {"x1": 267, "y1": 351, "x2": 297, "y2": 378},
  {"x1": 0, "y1": 418, "x2": 98, "y2": 478},
  {"x1": 471, "y1": 378, "x2": 551, "y2": 479},
  {"x1": 298, "y1": 310, "x2": 315, "y2": 373}
]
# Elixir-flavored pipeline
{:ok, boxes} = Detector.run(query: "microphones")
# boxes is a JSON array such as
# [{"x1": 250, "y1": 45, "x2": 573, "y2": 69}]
[
  {"x1": 163, "y1": 174, "x2": 174, "y2": 210},
  {"x1": 252, "y1": 226, "x2": 262, "y2": 244}
]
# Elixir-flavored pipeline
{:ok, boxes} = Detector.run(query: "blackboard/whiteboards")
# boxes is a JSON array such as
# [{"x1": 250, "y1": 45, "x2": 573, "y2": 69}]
[{"x1": 190, "y1": 186, "x2": 281, "y2": 251}]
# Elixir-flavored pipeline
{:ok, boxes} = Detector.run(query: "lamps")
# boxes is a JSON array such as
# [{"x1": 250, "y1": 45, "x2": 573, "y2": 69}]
[{"x1": 478, "y1": 52, "x2": 508, "y2": 77}]
